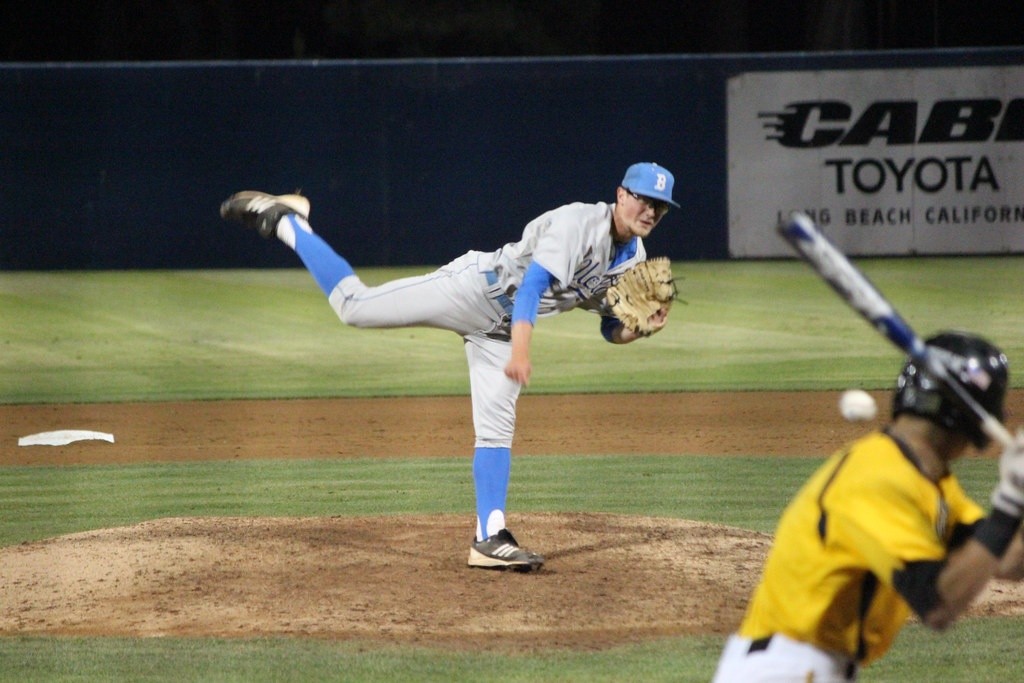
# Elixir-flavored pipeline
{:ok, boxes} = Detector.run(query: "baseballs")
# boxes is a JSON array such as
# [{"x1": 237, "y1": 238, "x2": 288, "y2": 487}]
[{"x1": 839, "y1": 390, "x2": 877, "y2": 424}]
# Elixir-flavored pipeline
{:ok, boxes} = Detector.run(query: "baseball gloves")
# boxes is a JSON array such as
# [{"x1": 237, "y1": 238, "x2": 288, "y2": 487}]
[{"x1": 606, "y1": 256, "x2": 678, "y2": 337}]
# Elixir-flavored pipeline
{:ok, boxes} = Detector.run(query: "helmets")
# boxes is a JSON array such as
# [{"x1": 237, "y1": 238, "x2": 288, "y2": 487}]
[{"x1": 890, "y1": 331, "x2": 1008, "y2": 452}]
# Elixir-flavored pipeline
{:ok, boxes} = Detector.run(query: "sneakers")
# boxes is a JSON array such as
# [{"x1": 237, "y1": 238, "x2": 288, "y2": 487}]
[
  {"x1": 468, "y1": 528, "x2": 544, "y2": 572},
  {"x1": 219, "y1": 190, "x2": 310, "y2": 240}
]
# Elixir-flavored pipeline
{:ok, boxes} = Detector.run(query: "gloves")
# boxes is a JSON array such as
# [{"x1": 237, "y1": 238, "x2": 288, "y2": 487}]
[{"x1": 990, "y1": 427, "x2": 1024, "y2": 516}]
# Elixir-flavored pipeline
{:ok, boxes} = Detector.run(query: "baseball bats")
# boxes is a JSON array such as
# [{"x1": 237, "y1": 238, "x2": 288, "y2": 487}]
[{"x1": 777, "y1": 210, "x2": 1013, "y2": 448}]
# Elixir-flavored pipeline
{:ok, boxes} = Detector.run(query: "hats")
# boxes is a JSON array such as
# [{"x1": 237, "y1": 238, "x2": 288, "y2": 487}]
[{"x1": 622, "y1": 162, "x2": 681, "y2": 209}]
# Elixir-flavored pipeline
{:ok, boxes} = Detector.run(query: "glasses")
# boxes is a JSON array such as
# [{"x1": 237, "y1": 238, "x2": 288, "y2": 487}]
[{"x1": 626, "y1": 190, "x2": 670, "y2": 215}]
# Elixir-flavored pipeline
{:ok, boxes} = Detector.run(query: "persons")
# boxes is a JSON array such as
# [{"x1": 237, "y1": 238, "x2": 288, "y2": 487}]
[
  {"x1": 220, "y1": 162, "x2": 681, "y2": 571},
  {"x1": 712, "y1": 331, "x2": 1024, "y2": 683}
]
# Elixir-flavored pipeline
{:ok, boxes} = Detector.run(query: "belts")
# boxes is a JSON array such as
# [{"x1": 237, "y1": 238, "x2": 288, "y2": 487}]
[{"x1": 487, "y1": 271, "x2": 515, "y2": 314}]
